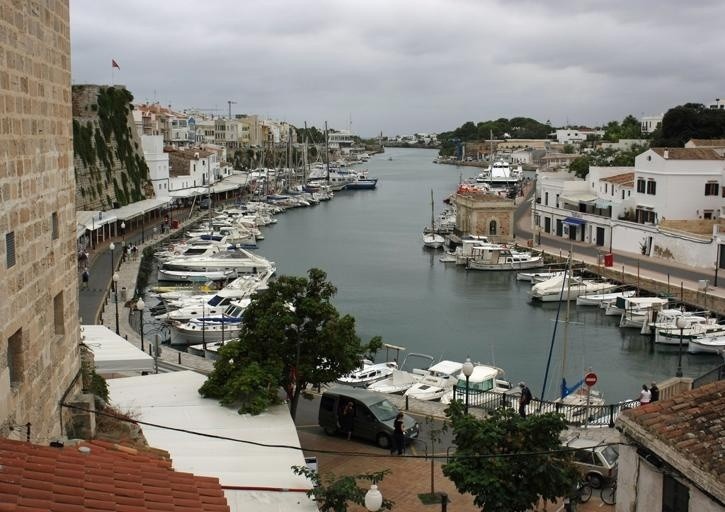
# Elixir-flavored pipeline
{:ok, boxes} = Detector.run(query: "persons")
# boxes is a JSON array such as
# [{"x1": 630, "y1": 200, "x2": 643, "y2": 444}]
[
  {"x1": 518, "y1": 381, "x2": 531, "y2": 417},
  {"x1": 641, "y1": 383, "x2": 652, "y2": 407},
  {"x1": 78, "y1": 245, "x2": 89, "y2": 263},
  {"x1": 81, "y1": 267, "x2": 90, "y2": 289},
  {"x1": 389, "y1": 411, "x2": 409, "y2": 455},
  {"x1": 650, "y1": 381, "x2": 661, "y2": 399},
  {"x1": 343, "y1": 401, "x2": 353, "y2": 441},
  {"x1": 121, "y1": 241, "x2": 139, "y2": 261}
]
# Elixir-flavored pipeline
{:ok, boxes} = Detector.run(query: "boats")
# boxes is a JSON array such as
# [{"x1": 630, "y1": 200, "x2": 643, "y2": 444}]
[
  {"x1": 402, "y1": 360, "x2": 464, "y2": 402},
  {"x1": 495, "y1": 395, "x2": 541, "y2": 416},
  {"x1": 440, "y1": 348, "x2": 513, "y2": 410},
  {"x1": 337, "y1": 360, "x2": 398, "y2": 387},
  {"x1": 366, "y1": 353, "x2": 434, "y2": 396},
  {"x1": 581, "y1": 400, "x2": 641, "y2": 428}
]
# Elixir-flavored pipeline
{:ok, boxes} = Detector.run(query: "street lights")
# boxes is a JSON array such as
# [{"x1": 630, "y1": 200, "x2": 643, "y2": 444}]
[
  {"x1": 120, "y1": 221, "x2": 126, "y2": 262},
  {"x1": 109, "y1": 242, "x2": 115, "y2": 292},
  {"x1": 364, "y1": 479, "x2": 383, "y2": 511},
  {"x1": 112, "y1": 272, "x2": 120, "y2": 336},
  {"x1": 462, "y1": 353, "x2": 474, "y2": 417},
  {"x1": 140, "y1": 209, "x2": 145, "y2": 244},
  {"x1": 673, "y1": 309, "x2": 687, "y2": 377},
  {"x1": 136, "y1": 298, "x2": 148, "y2": 375}
]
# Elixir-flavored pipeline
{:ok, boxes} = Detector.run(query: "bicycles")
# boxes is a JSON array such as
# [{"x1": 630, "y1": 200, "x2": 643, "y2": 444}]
[{"x1": 600, "y1": 477, "x2": 617, "y2": 507}]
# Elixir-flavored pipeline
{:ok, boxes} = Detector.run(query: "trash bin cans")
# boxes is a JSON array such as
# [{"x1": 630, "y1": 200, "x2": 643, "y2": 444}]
[
  {"x1": 121, "y1": 287, "x2": 126, "y2": 302},
  {"x1": 304, "y1": 456, "x2": 318, "y2": 487}
]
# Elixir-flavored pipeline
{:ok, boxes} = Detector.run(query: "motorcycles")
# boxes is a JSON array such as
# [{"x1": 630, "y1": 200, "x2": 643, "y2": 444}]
[{"x1": 565, "y1": 477, "x2": 593, "y2": 511}]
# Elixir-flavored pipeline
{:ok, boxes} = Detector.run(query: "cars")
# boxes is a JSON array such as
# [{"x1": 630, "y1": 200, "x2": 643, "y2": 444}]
[{"x1": 560, "y1": 435, "x2": 619, "y2": 489}]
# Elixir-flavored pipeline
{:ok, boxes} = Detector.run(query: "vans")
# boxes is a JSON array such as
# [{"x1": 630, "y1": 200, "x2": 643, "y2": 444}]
[{"x1": 319, "y1": 388, "x2": 419, "y2": 449}]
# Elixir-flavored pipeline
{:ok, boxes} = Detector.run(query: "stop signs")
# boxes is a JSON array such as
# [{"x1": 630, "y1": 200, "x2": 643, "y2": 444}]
[{"x1": 585, "y1": 373, "x2": 597, "y2": 387}]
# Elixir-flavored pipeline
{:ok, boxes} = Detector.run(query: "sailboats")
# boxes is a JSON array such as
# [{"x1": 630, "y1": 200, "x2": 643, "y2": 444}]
[{"x1": 533, "y1": 248, "x2": 605, "y2": 425}]
